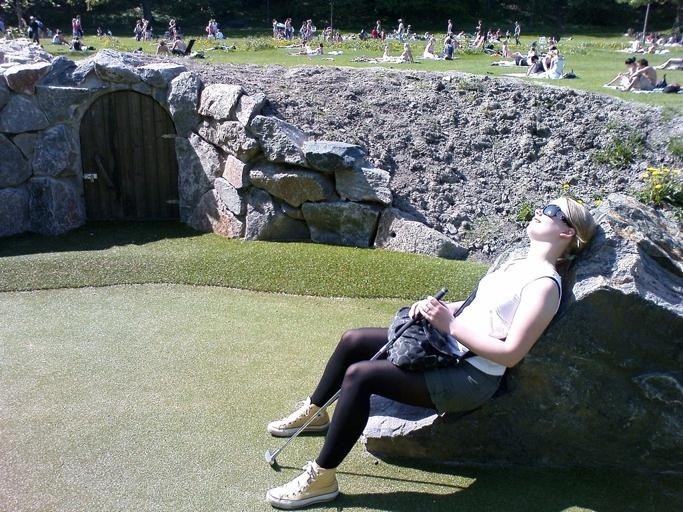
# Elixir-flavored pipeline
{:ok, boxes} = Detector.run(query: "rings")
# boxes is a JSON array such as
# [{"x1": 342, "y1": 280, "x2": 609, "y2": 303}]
[{"x1": 425, "y1": 308, "x2": 429, "y2": 313}]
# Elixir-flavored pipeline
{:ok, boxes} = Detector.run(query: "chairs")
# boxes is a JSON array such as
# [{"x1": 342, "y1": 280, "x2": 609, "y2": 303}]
[{"x1": 172, "y1": 39, "x2": 196, "y2": 55}]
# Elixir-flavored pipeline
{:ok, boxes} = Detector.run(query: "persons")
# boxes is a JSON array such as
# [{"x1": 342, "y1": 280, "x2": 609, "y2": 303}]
[
  {"x1": 602, "y1": 24, "x2": 682, "y2": 92},
  {"x1": 1, "y1": 13, "x2": 566, "y2": 80},
  {"x1": 264, "y1": 196, "x2": 597, "y2": 511}
]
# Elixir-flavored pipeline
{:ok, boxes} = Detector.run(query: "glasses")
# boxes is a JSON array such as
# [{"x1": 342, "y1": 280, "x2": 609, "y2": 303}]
[{"x1": 540, "y1": 203, "x2": 573, "y2": 229}]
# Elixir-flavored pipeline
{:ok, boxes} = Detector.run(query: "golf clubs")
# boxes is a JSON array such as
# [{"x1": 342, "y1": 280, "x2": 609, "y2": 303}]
[{"x1": 265, "y1": 288, "x2": 448, "y2": 465}]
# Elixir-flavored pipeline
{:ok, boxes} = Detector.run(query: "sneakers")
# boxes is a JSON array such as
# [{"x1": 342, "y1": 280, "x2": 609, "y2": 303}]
[
  {"x1": 265, "y1": 459, "x2": 340, "y2": 510},
  {"x1": 267, "y1": 395, "x2": 331, "y2": 438}
]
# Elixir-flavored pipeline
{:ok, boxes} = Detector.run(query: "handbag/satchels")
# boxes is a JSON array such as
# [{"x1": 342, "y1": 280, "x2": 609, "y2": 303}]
[{"x1": 388, "y1": 288, "x2": 479, "y2": 371}]
